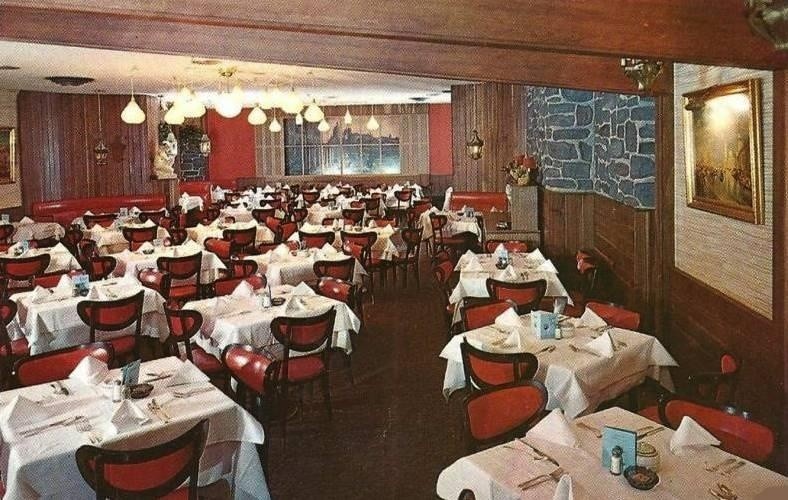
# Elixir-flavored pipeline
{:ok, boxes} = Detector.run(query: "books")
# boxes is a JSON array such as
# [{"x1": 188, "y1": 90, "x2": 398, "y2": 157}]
[
  {"x1": 120, "y1": 358, "x2": 141, "y2": 386},
  {"x1": 602, "y1": 425, "x2": 636, "y2": 472},
  {"x1": 530, "y1": 309, "x2": 556, "y2": 338}
]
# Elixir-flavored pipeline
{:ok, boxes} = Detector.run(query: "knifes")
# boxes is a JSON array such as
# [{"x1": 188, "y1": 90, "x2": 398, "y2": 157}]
[
  {"x1": 637, "y1": 427, "x2": 664, "y2": 442},
  {"x1": 56, "y1": 379, "x2": 69, "y2": 397},
  {"x1": 145, "y1": 373, "x2": 174, "y2": 383},
  {"x1": 101, "y1": 282, "x2": 118, "y2": 286},
  {"x1": 515, "y1": 437, "x2": 559, "y2": 468},
  {"x1": 533, "y1": 345, "x2": 550, "y2": 356}
]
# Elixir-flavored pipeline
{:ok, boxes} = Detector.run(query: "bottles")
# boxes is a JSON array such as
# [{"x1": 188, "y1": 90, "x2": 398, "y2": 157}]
[
  {"x1": 554, "y1": 326, "x2": 561, "y2": 340},
  {"x1": 263, "y1": 293, "x2": 271, "y2": 309},
  {"x1": 113, "y1": 380, "x2": 122, "y2": 402},
  {"x1": 610, "y1": 448, "x2": 623, "y2": 475}
]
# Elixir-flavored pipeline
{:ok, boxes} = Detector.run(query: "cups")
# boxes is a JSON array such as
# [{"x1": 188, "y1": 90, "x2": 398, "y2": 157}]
[
  {"x1": 328, "y1": 201, "x2": 334, "y2": 209},
  {"x1": 2, "y1": 215, "x2": 10, "y2": 225},
  {"x1": 120, "y1": 208, "x2": 128, "y2": 217},
  {"x1": 153, "y1": 239, "x2": 163, "y2": 250}
]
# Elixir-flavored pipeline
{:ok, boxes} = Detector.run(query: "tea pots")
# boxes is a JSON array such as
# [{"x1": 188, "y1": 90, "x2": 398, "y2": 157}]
[{"x1": 495, "y1": 220, "x2": 509, "y2": 228}]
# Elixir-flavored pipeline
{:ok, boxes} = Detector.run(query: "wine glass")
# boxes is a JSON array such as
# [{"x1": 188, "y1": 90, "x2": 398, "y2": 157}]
[{"x1": 456, "y1": 210, "x2": 464, "y2": 224}]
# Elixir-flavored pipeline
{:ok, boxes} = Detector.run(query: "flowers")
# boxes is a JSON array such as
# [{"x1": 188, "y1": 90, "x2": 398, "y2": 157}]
[{"x1": 501, "y1": 151, "x2": 537, "y2": 177}]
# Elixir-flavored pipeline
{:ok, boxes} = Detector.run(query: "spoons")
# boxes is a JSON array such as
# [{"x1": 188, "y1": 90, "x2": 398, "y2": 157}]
[
  {"x1": 706, "y1": 457, "x2": 735, "y2": 472},
  {"x1": 521, "y1": 472, "x2": 569, "y2": 491},
  {"x1": 20, "y1": 416, "x2": 82, "y2": 437},
  {"x1": 503, "y1": 446, "x2": 546, "y2": 461},
  {"x1": 151, "y1": 399, "x2": 170, "y2": 420},
  {"x1": 147, "y1": 367, "x2": 190, "y2": 375}
]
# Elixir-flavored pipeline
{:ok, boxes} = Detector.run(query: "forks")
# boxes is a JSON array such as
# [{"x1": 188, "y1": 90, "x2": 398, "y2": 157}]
[
  {"x1": 173, "y1": 388, "x2": 214, "y2": 399},
  {"x1": 578, "y1": 422, "x2": 602, "y2": 439},
  {"x1": 569, "y1": 343, "x2": 601, "y2": 358},
  {"x1": 517, "y1": 467, "x2": 561, "y2": 488},
  {"x1": 718, "y1": 461, "x2": 744, "y2": 476},
  {"x1": 147, "y1": 402, "x2": 168, "y2": 424}
]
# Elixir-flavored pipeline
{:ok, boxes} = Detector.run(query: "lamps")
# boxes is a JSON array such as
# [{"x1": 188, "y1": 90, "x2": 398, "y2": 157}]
[
  {"x1": 343, "y1": 106, "x2": 352, "y2": 125},
  {"x1": 366, "y1": 105, "x2": 380, "y2": 130},
  {"x1": 620, "y1": 58, "x2": 663, "y2": 93},
  {"x1": 91, "y1": 92, "x2": 110, "y2": 166},
  {"x1": 198, "y1": 115, "x2": 211, "y2": 159},
  {"x1": 120, "y1": 78, "x2": 146, "y2": 124},
  {"x1": 158, "y1": 67, "x2": 330, "y2": 132},
  {"x1": 464, "y1": 83, "x2": 484, "y2": 159}
]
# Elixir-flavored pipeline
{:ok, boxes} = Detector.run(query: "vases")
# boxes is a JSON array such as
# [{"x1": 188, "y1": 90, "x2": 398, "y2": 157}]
[{"x1": 515, "y1": 173, "x2": 531, "y2": 184}]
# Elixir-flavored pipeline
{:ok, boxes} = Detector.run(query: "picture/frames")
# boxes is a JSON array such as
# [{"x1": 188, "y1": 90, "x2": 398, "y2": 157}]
[
  {"x1": 681, "y1": 78, "x2": 764, "y2": 226},
  {"x1": 0, "y1": 127, "x2": 16, "y2": 185}
]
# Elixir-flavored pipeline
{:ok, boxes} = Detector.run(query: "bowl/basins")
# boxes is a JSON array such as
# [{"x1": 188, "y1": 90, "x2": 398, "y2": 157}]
[
  {"x1": 496, "y1": 264, "x2": 508, "y2": 269},
  {"x1": 131, "y1": 384, "x2": 153, "y2": 398},
  {"x1": 332, "y1": 227, "x2": 342, "y2": 232},
  {"x1": 143, "y1": 249, "x2": 155, "y2": 255},
  {"x1": 354, "y1": 227, "x2": 362, "y2": 231},
  {"x1": 623, "y1": 466, "x2": 659, "y2": 490},
  {"x1": 291, "y1": 251, "x2": 298, "y2": 256},
  {"x1": 273, "y1": 296, "x2": 284, "y2": 305}
]
